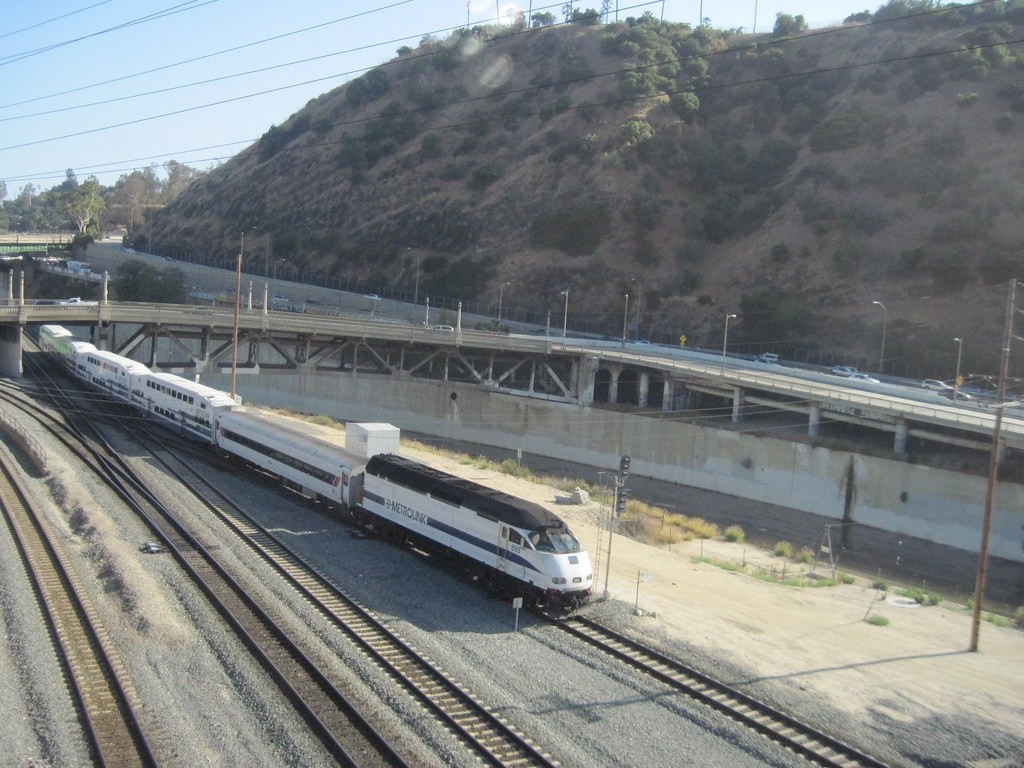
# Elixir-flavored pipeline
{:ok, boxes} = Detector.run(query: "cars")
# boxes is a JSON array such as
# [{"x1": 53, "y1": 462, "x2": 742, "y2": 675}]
[
  {"x1": 848, "y1": 373, "x2": 881, "y2": 384},
  {"x1": 831, "y1": 364, "x2": 859, "y2": 377},
  {"x1": 937, "y1": 388, "x2": 971, "y2": 401},
  {"x1": 274, "y1": 295, "x2": 290, "y2": 302},
  {"x1": 363, "y1": 293, "x2": 381, "y2": 301},
  {"x1": 66, "y1": 297, "x2": 79, "y2": 304},
  {"x1": 920, "y1": 379, "x2": 954, "y2": 392}
]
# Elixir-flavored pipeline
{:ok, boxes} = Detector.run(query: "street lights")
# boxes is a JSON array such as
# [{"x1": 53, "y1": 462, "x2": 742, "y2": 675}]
[
  {"x1": 621, "y1": 294, "x2": 628, "y2": 348},
  {"x1": 952, "y1": 336, "x2": 964, "y2": 404},
  {"x1": 872, "y1": 299, "x2": 888, "y2": 381},
  {"x1": 561, "y1": 291, "x2": 569, "y2": 337},
  {"x1": 720, "y1": 314, "x2": 737, "y2": 375},
  {"x1": 497, "y1": 282, "x2": 511, "y2": 331},
  {"x1": 253, "y1": 225, "x2": 269, "y2": 286},
  {"x1": 269, "y1": 258, "x2": 286, "y2": 310},
  {"x1": 631, "y1": 277, "x2": 642, "y2": 345},
  {"x1": 407, "y1": 246, "x2": 420, "y2": 326}
]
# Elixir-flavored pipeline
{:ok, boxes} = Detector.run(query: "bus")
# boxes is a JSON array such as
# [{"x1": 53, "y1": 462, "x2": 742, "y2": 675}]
[{"x1": 67, "y1": 260, "x2": 91, "y2": 274}]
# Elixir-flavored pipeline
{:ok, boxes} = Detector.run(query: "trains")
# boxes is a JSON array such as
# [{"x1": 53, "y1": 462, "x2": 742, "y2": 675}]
[{"x1": 36, "y1": 321, "x2": 595, "y2": 611}]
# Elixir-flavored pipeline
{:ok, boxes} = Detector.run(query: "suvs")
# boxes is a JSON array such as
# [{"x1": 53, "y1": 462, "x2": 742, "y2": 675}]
[
  {"x1": 33, "y1": 299, "x2": 70, "y2": 312},
  {"x1": 430, "y1": 324, "x2": 455, "y2": 332}
]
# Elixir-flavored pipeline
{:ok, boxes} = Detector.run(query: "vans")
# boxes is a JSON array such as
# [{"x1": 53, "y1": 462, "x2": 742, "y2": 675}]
[{"x1": 753, "y1": 351, "x2": 782, "y2": 364}]
